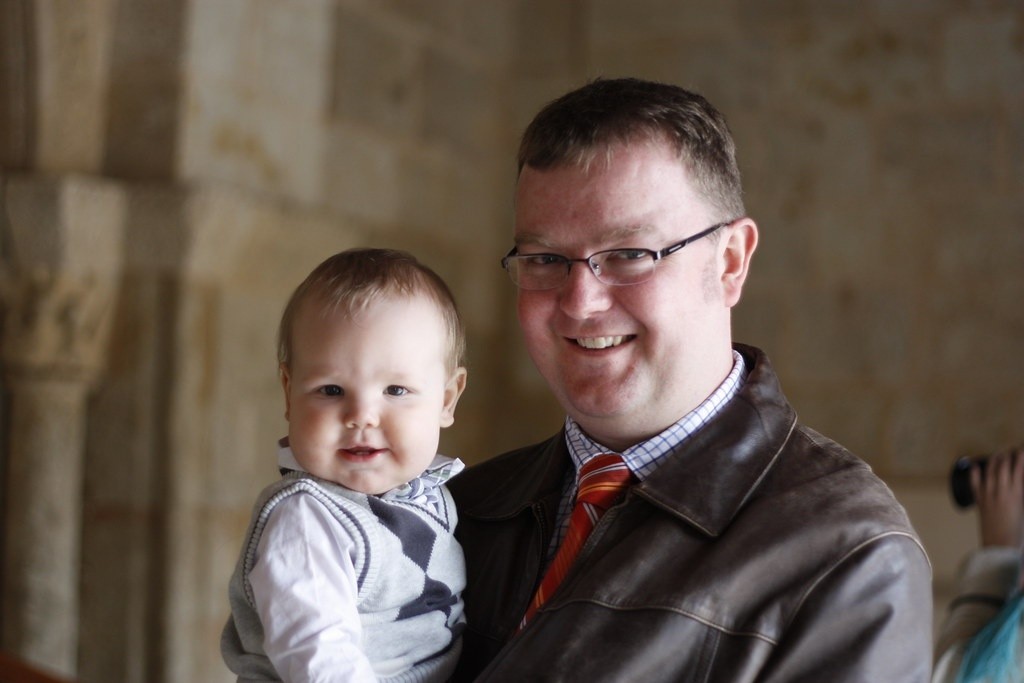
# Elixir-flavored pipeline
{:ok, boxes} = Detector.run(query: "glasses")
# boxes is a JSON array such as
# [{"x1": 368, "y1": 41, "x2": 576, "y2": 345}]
[{"x1": 501, "y1": 221, "x2": 734, "y2": 291}]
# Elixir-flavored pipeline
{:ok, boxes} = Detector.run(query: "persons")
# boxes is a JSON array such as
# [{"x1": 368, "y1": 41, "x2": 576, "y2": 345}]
[
  {"x1": 219, "y1": 248, "x2": 467, "y2": 683},
  {"x1": 444, "y1": 76, "x2": 934, "y2": 683},
  {"x1": 938, "y1": 445, "x2": 1024, "y2": 683}
]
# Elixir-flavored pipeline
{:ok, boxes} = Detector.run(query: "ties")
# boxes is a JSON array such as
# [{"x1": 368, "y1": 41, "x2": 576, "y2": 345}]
[{"x1": 513, "y1": 454, "x2": 635, "y2": 637}]
